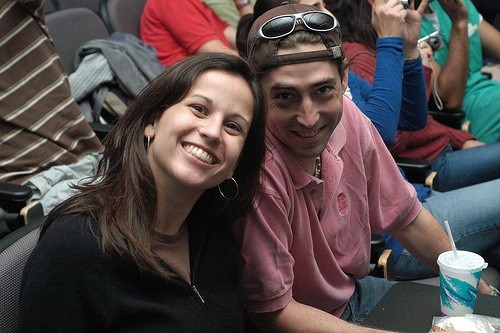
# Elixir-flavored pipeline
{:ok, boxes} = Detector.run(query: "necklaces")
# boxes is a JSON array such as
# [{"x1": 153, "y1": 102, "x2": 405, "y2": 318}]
[{"x1": 314, "y1": 156, "x2": 321, "y2": 177}]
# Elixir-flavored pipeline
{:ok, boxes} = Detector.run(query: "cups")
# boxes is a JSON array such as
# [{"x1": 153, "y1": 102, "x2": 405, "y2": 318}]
[{"x1": 436, "y1": 250, "x2": 489, "y2": 316}]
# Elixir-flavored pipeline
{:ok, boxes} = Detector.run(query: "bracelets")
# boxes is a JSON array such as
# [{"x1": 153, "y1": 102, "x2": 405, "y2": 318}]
[{"x1": 490, "y1": 289, "x2": 497, "y2": 295}]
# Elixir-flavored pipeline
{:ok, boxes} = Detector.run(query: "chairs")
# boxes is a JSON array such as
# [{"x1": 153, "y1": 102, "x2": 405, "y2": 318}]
[
  {"x1": 44, "y1": 0, "x2": 146, "y2": 76},
  {"x1": 0, "y1": 215, "x2": 47, "y2": 333}
]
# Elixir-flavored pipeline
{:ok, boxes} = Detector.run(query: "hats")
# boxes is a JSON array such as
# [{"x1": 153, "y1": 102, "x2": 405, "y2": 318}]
[{"x1": 247, "y1": 0, "x2": 345, "y2": 74}]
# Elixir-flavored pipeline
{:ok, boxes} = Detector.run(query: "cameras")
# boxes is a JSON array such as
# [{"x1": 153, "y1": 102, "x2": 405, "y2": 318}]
[
  {"x1": 384, "y1": 0, "x2": 409, "y2": 10},
  {"x1": 417, "y1": 30, "x2": 445, "y2": 52}
]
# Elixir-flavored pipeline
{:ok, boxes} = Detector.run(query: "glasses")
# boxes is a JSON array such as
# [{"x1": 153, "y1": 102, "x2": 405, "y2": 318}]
[{"x1": 248, "y1": 9, "x2": 342, "y2": 62}]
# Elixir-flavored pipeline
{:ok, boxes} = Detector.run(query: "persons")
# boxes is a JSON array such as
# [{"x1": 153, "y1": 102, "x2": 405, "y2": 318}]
[
  {"x1": 0, "y1": 0, "x2": 499, "y2": 280},
  {"x1": 231, "y1": 4, "x2": 499, "y2": 332},
  {"x1": 19, "y1": 52, "x2": 269, "y2": 333}
]
